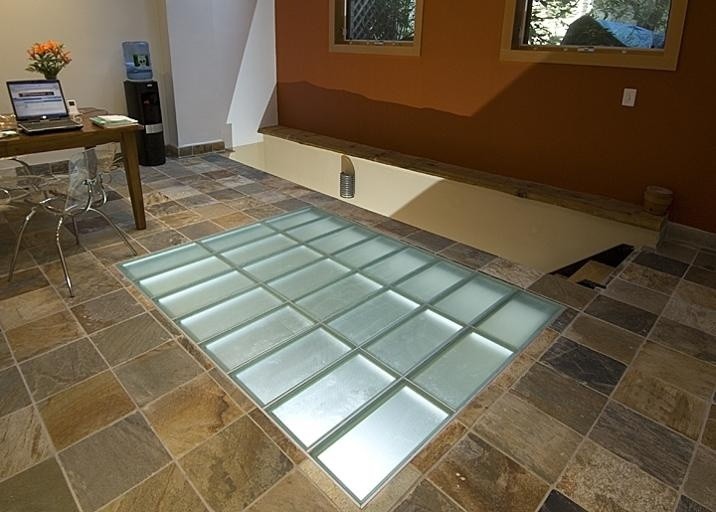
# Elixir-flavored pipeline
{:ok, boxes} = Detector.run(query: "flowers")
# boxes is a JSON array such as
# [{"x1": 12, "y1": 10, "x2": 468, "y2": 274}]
[{"x1": 23, "y1": 39, "x2": 73, "y2": 79}]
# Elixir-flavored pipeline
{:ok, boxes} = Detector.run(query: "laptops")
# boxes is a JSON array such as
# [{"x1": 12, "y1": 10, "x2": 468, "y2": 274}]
[{"x1": 6, "y1": 80, "x2": 84, "y2": 135}]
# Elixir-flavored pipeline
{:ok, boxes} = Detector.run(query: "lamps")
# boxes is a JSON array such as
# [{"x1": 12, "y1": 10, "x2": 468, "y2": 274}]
[{"x1": 340, "y1": 172, "x2": 355, "y2": 198}]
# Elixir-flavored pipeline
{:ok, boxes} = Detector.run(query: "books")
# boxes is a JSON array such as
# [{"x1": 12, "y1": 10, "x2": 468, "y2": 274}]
[{"x1": 90, "y1": 113, "x2": 137, "y2": 129}]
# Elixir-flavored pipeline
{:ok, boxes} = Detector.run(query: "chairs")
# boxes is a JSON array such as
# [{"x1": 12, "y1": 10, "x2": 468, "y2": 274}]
[{"x1": 0, "y1": 142, "x2": 138, "y2": 297}]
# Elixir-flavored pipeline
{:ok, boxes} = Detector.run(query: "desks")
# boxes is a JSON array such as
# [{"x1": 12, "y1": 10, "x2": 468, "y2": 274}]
[{"x1": 0, "y1": 107, "x2": 146, "y2": 230}]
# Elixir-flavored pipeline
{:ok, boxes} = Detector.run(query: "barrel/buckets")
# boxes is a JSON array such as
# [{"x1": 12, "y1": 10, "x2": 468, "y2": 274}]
[{"x1": 121, "y1": 41, "x2": 154, "y2": 81}]
